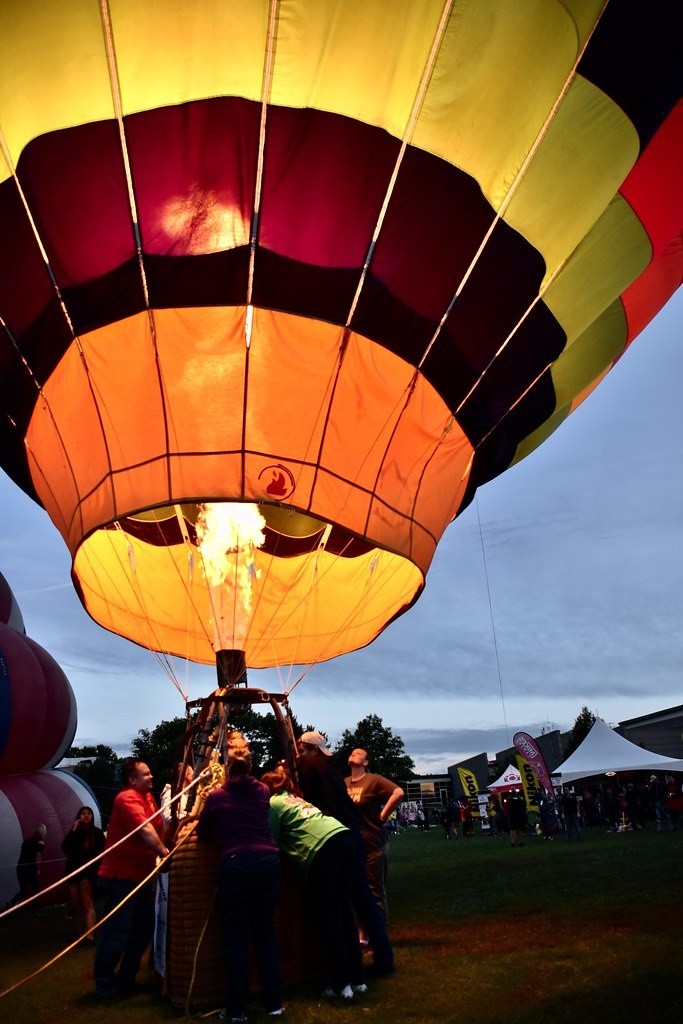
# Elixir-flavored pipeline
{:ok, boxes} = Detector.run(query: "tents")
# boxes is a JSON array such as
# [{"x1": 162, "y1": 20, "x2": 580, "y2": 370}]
[
  {"x1": 550, "y1": 717, "x2": 683, "y2": 784},
  {"x1": 485, "y1": 764, "x2": 523, "y2": 791}
]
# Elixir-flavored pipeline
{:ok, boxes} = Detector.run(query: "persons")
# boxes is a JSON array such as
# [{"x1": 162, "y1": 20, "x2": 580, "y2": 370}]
[
  {"x1": 403, "y1": 788, "x2": 528, "y2": 847},
  {"x1": 62, "y1": 806, "x2": 106, "y2": 941},
  {"x1": 95, "y1": 723, "x2": 404, "y2": 1024},
  {"x1": 6, "y1": 823, "x2": 46, "y2": 913},
  {"x1": 536, "y1": 775, "x2": 683, "y2": 841}
]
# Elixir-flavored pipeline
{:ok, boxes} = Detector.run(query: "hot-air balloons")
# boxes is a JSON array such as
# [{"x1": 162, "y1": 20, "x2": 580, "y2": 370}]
[
  {"x1": 0, "y1": 1, "x2": 682, "y2": 1013},
  {"x1": 0, "y1": 568, "x2": 107, "y2": 933}
]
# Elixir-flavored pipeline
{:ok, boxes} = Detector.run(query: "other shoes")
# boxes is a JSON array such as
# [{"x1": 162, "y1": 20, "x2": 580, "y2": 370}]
[
  {"x1": 512, "y1": 843, "x2": 516, "y2": 847},
  {"x1": 82, "y1": 940, "x2": 96, "y2": 946},
  {"x1": 518, "y1": 843, "x2": 524, "y2": 847},
  {"x1": 218, "y1": 1007, "x2": 248, "y2": 1024},
  {"x1": 322, "y1": 982, "x2": 355, "y2": 1004},
  {"x1": 350, "y1": 982, "x2": 369, "y2": 993},
  {"x1": 96, "y1": 984, "x2": 125, "y2": 999},
  {"x1": 263, "y1": 1001, "x2": 286, "y2": 1020},
  {"x1": 367, "y1": 967, "x2": 396, "y2": 979},
  {"x1": 32, "y1": 910, "x2": 46, "y2": 915}
]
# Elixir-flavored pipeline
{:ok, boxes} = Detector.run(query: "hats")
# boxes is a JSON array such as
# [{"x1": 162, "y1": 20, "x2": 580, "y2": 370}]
[
  {"x1": 650, "y1": 775, "x2": 657, "y2": 783},
  {"x1": 300, "y1": 731, "x2": 333, "y2": 756}
]
